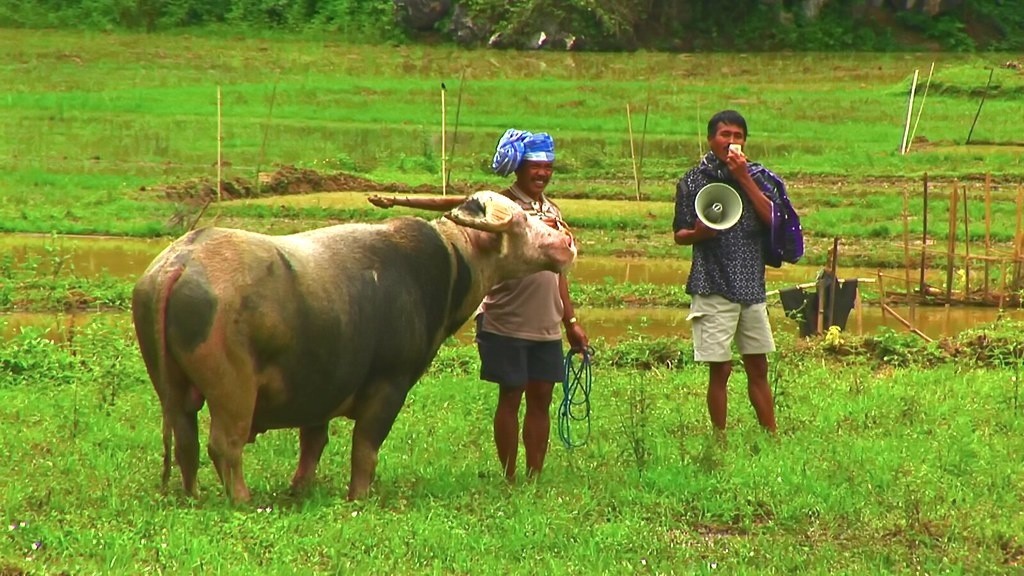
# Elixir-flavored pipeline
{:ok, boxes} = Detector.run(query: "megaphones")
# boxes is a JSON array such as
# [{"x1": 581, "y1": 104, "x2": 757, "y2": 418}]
[{"x1": 694, "y1": 183, "x2": 742, "y2": 229}]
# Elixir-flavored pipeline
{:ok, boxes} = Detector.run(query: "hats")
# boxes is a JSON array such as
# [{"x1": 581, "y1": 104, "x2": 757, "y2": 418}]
[{"x1": 492, "y1": 129, "x2": 556, "y2": 178}]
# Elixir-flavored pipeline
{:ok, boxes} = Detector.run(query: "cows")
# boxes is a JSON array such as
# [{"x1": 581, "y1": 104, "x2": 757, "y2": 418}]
[{"x1": 128, "y1": 184, "x2": 581, "y2": 513}]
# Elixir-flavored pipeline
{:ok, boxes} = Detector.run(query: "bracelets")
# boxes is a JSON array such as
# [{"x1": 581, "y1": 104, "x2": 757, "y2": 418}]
[{"x1": 563, "y1": 316, "x2": 577, "y2": 327}]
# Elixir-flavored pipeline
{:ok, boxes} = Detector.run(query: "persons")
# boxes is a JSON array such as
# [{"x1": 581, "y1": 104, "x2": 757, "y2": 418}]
[
  {"x1": 474, "y1": 127, "x2": 589, "y2": 487},
  {"x1": 673, "y1": 109, "x2": 780, "y2": 455}
]
